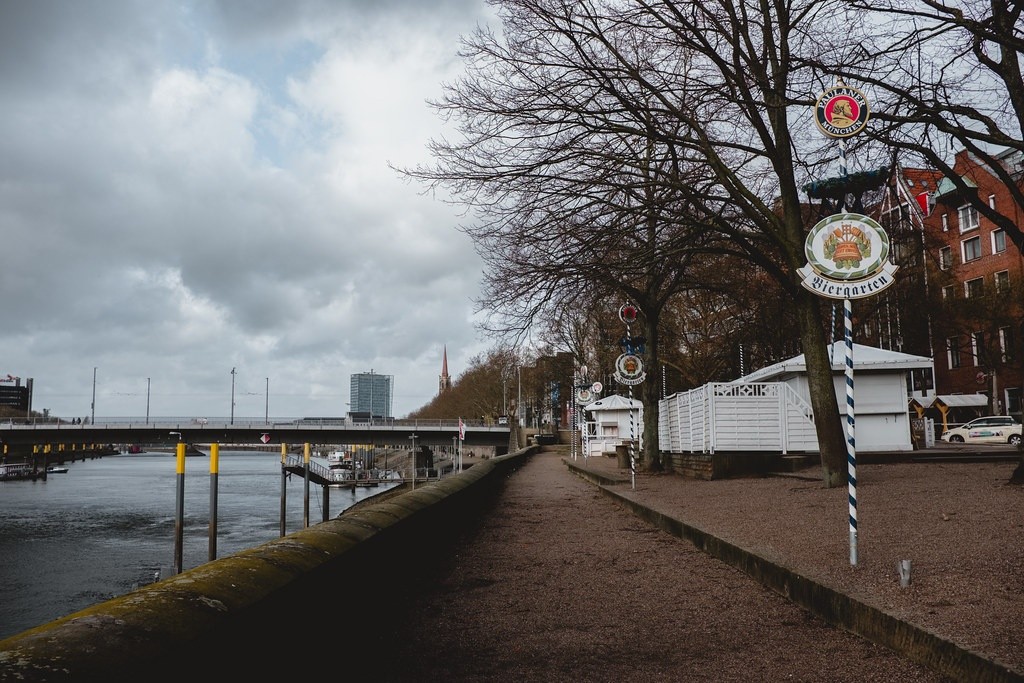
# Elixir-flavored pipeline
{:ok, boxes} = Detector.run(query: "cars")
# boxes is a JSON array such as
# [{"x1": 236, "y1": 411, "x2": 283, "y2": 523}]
[
  {"x1": 942, "y1": 415, "x2": 1023, "y2": 444},
  {"x1": 499, "y1": 417, "x2": 508, "y2": 425}
]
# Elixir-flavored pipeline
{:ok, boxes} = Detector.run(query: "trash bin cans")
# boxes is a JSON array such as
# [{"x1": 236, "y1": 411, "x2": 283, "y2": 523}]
[{"x1": 616, "y1": 445, "x2": 631, "y2": 468}]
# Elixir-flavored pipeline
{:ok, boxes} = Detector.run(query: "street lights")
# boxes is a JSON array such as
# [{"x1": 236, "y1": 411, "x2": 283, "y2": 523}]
[
  {"x1": 92, "y1": 367, "x2": 98, "y2": 425},
  {"x1": 265, "y1": 377, "x2": 269, "y2": 426}
]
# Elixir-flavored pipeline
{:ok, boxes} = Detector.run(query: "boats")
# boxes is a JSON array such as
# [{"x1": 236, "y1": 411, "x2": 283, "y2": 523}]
[
  {"x1": 47, "y1": 466, "x2": 69, "y2": 473},
  {"x1": 174, "y1": 443, "x2": 206, "y2": 456},
  {"x1": 327, "y1": 457, "x2": 381, "y2": 486},
  {"x1": 146, "y1": 378, "x2": 151, "y2": 423},
  {"x1": 128, "y1": 446, "x2": 146, "y2": 453}
]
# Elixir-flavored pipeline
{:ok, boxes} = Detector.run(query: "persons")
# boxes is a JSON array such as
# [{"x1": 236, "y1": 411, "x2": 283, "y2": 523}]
[
  {"x1": 72, "y1": 417, "x2": 81, "y2": 425},
  {"x1": 480, "y1": 416, "x2": 485, "y2": 425}
]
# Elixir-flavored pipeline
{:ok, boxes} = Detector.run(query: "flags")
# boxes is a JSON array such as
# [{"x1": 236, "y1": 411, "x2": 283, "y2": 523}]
[{"x1": 459, "y1": 418, "x2": 466, "y2": 441}]
